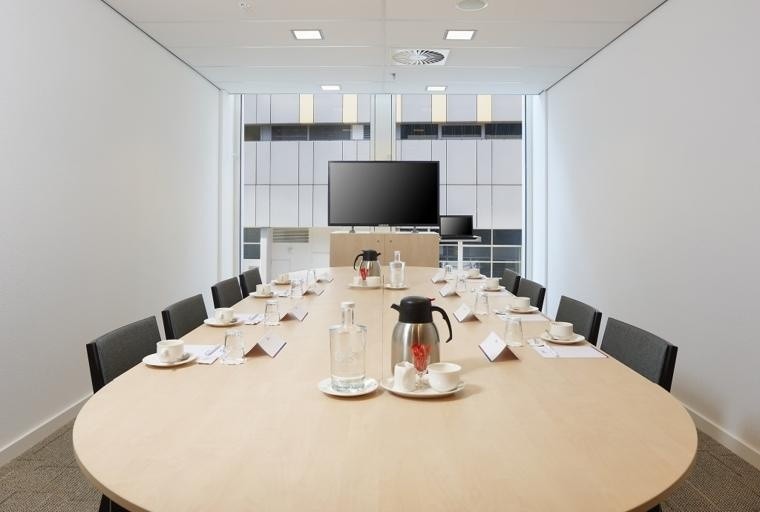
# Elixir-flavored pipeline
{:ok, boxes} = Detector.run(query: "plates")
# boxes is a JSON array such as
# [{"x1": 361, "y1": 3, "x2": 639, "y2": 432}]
[
  {"x1": 318, "y1": 377, "x2": 378, "y2": 397},
  {"x1": 506, "y1": 306, "x2": 538, "y2": 313},
  {"x1": 273, "y1": 279, "x2": 291, "y2": 285},
  {"x1": 384, "y1": 283, "x2": 407, "y2": 289},
  {"x1": 541, "y1": 333, "x2": 586, "y2": 345},
  {"x1": 142, "y1": 352, "x2": 197, "y2": 367},
  {"x1": 348, "y1": 283, "x2": 381, "y2": 289},
  {"x1": 203, "y1": 319, "x2": 240, "y2": 327},
  {"x1": 480, "y1": 286, "x2": 505, "y2": 292},
  {"x1": 467, "y1": 276, "x2": 483, "y2": 280},
  {"x1": 250, "y1": 292, "x2": 273, "y2": 297},
  {"x1": 379, "y1": 376, "x2": 464, "y2": 398}
]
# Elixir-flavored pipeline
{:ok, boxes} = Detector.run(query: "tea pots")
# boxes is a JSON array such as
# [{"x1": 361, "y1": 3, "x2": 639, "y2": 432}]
[
  {"x1": 353, "y1": 249, "x2": 384, "y2": 280},
  {"x1": 390, "y1": 297, "x2": 452, "y2": 371}
]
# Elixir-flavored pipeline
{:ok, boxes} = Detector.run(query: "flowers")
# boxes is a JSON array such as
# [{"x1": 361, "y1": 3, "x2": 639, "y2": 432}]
[
  {"x1": 360, "y1": 266, "x2": 369, "y2": 280},
  {"x1": 410, "y1": 343, "x2": 432, "y2": 371}
]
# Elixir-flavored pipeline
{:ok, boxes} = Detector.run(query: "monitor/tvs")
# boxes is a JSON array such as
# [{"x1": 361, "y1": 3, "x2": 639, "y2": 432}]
[{"x1": 329, "y1": 160, "x2": 441, "y2": 231}]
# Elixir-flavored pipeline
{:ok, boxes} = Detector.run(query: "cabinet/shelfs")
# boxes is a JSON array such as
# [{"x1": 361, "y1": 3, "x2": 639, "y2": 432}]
[{"x1": 329, "y1": 230, "x2": 440, "y2": 268}]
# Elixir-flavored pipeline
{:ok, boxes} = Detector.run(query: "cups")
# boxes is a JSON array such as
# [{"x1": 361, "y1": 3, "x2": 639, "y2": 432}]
[
  {"x1": 456, "y1": 274, "x2": 466, "y2": 290},
  {"x1": 307, "y1": 269, "x2": 317, "y2": 284},
  {"x1": 470, "y1": 269, "x2": 480, "y2": 277},
  {"x1": 353, "y1": 276, "x2": 362, "y2": 285},
  {"x1": 222, "y1": 330, "x2": 244, "y2": 364},
  {"x1": 255, "y1": 285, "x2": 270, "y2": 294},
  {"x1": 445, "y1": 265, "x2": 453, "y2": 279},
  {"x1": 291, "y1": 280, "x2": 303, "y2": 299},
  {"x1": 156, "y1": 340, "x2": 185, "y2": 362},
  {"x1": 504, "y1": 316, "x2": 524, "y2": 347},
  {"x1": 474, "y1": 292, "x2": 489, "y2": 316},
  {"x1": 512, "y1": 296, "x2": 530, "y2": 310},
  {"x1": 550, "y1": 322, "x2": 574, "y2": 339},
  {"x1": 482, "y1": 278, "x2": 499, "y2": 289},
  {"x1": 212, "y1": 308, "x2": 234, "y2": 321},
  {"x1": 277, "y1": 271, "x2": 289, "y2": 282},
  {"x1": 427, "y1": 362, "x2": 461, "y2": 392},
  {"x1": 394, "y1": 361, "x2": 416, "y2": 391},
  {"x1": 366, "y1": 276, "x2": 380, "y2": 287},
  {"x1": 264, "y1": 301, "x2": 281, "y2": 326}
]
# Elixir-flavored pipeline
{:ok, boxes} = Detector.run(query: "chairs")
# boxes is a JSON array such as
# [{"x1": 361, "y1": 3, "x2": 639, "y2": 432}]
[
  {"x1": 555, "y1": 295, "x2": 602, "y2": 347},
  {"x1": 500, "y1": 268, "x2": 520, "y2": 294},
  {"x1": 85, "y1": 315, "x2": 161, "y2": 512},
  {"x1": 516, "y1": 278, "x2": 546, "y2": 313},
  {"x1": 161, "y1": 293, "x2": 208, "y2": 339},
  {"x1": 210, "y1": 277, "x2": 243, "y2": 308},
  {"x1": 239, "y1": 266, "x2": 262, "y2": 298},
  {"x1": 600, "y1": 317, "x2": 678, "y2": 512}
]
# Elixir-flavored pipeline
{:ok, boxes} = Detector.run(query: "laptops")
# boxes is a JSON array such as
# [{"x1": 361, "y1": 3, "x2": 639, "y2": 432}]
[{"x1": 438, "y1": 215, "x2": 476, "y2": 239}]
